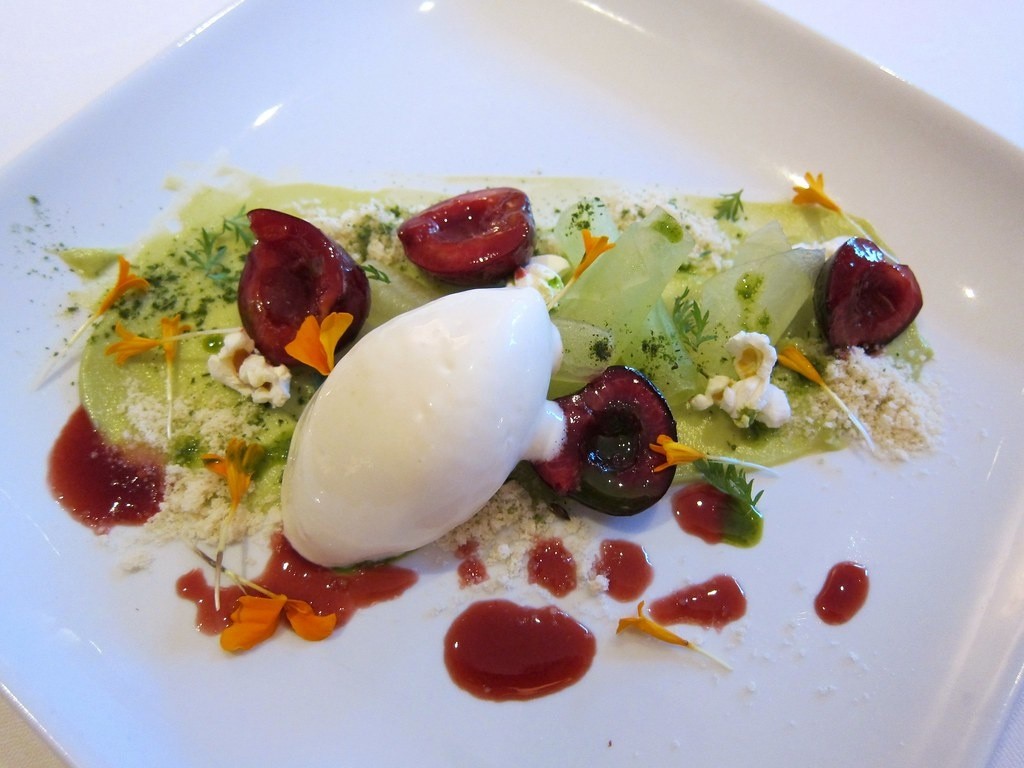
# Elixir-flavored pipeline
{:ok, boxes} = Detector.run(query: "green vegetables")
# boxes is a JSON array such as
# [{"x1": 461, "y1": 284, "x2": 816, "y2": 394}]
[{"x1": 182, "y1": 188, "x2": 763, "y2": 515}]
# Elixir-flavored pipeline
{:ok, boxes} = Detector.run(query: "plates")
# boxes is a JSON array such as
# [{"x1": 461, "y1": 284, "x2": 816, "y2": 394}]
[{"x1": 0, "y1": 0, "x2": 1023, "y2": 768}]
